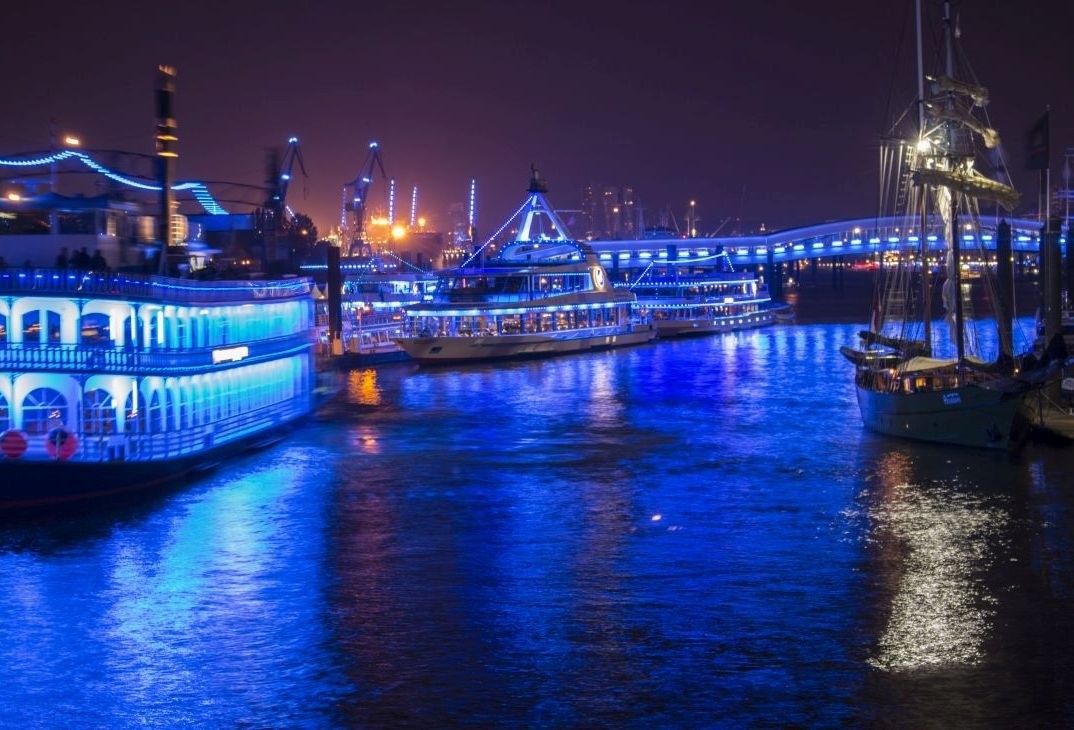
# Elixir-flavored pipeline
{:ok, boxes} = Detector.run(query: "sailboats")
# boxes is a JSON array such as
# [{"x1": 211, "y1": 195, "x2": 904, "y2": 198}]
[{"x1": 839, "y1": 0, "x2": 1044, "y2": 450}]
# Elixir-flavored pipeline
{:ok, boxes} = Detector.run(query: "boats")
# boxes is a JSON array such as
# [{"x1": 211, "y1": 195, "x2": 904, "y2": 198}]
[
  {"x1": 317, "y1": 237, "x2": 791, "y2": 370},
  {"x1": 0, "y1": 254, "x2": 329, "y2": 505}
]
